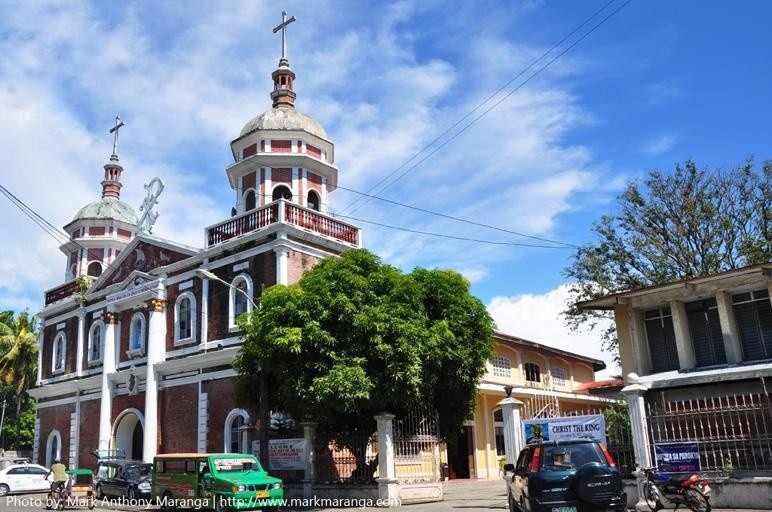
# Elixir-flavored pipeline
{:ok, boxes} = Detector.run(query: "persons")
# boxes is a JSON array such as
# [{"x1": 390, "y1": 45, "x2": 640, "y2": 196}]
[
  {"x1": 44, "y1": 456, "x2": 69, "y2": 509},
  {"x1": 524, "y1": 427, "x2": 549, "y2": 445}
]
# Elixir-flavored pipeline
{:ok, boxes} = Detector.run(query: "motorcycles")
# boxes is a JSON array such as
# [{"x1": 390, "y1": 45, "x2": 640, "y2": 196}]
[{"x1": 640, "y1": 465, "x2": 711, "y2": 512}]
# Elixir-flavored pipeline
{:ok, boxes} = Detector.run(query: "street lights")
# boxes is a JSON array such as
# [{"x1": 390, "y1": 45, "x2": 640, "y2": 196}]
[{"x1": 194, "y1": 267, "x2": 273, "y2": 473}]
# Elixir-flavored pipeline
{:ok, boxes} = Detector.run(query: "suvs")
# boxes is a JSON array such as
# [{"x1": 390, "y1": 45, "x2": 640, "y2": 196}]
[{"x1": 502, "y1": 435, "x2": 627, "y2": 511}]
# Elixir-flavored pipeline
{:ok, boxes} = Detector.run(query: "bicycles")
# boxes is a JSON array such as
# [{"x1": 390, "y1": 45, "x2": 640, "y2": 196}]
[{"x1": 45, "y1": 478, "x2": 69, "y2": 510}]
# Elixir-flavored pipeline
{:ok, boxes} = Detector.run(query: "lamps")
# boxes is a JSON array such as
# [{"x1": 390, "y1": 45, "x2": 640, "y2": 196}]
[
  {"x1": 504, "y1": 384, "x2": 513, "y2": 398},
  {"x1": 134, "y1": 277, "x2": 140, "y2": 286}
]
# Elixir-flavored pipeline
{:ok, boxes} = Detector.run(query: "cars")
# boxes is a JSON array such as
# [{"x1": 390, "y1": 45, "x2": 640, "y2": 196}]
[
  {"x1": 0, "y1": 464, "x2": 70, "y2": 496},
  {"x1": 90, "y1": 448, "x2": 154, "y2": 504}
]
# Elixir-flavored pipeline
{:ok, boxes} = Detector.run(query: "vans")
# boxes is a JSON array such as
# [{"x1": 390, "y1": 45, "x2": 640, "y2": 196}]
[{"x1": 151, "y1": 452, "x2": 283, "y2": 512}]
